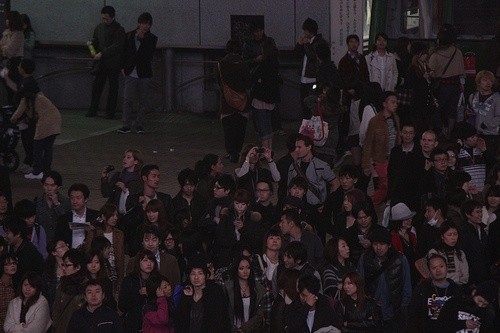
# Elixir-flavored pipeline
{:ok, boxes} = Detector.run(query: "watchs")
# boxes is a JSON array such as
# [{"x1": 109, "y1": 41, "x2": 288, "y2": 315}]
[{"x1": 245, "y1": 161, "x2": 248, "y2": 163}]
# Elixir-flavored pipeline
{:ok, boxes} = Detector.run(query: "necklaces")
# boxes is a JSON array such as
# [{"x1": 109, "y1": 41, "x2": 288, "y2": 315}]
[
  {"x1": 435, "y1": 280, "x2": 447, "y2": 297},
  {"x1": 58, "y1": 264, "x2": 62, "y2": 269},
  {"x1": 241, "y1": 288, "x2": 247, "y2": 296},
  {"x1": 268, "y1": 256, "x2": 277, "y2": 261}
]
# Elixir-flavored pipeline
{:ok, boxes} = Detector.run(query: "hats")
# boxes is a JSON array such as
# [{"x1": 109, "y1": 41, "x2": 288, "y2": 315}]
[{"x1": 389, "y1": 203, "x2": 416, "y2": 220}]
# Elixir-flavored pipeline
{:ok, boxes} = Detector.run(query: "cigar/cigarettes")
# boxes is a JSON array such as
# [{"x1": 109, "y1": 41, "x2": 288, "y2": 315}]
[{"x1": 256, "y1": 197, "x2": 261, "y2": 202}]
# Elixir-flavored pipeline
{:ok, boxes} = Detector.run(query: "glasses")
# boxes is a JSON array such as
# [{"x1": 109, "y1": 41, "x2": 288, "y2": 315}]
[
  {"x1": 163, "y1": 238, "x2": 174, "y2": 244},
  {"x1": 55, "y1": 244, "x2": 69, "y2": 249},
  {"x1": 213, "y1": 184, "x2": 225, "y2": 189},
  {"x1": 59, "y1": 263, "x2": 75, "y2": 269},
  {"x1": 4, "y1": 261, "x2": 19, "y2": 266}
]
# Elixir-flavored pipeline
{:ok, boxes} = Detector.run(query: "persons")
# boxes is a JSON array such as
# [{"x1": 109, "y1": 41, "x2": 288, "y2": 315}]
[
  {"x1": 9, "y1": 88, "x2": 62, "y2": 179},
  {"x1": 0, "y1": 11, "x2": 40, "y2": 173},
  {"x1": 0, "y1": 123, "x2": 500, "y2": 333},
  {"x1": 85, "y1": 6, "x2": 125, "y2": 119},
  {"x1": 222, "y1": 17, "x2": 465, "y2": 158},
  {"x1": 464, "y1": 70, "x2": 500, "y2": 178},
  {"x1": 118, "y1": 12, "x2": 158, "y2": 133},
  {"x1": 365, "y1": 91, "x2": 401, "y2": 191}
]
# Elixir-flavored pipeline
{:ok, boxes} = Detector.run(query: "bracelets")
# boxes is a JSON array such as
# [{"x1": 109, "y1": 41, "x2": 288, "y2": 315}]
[{"x1": 268, "y1": 160, "x2": 273, "y2": 163}]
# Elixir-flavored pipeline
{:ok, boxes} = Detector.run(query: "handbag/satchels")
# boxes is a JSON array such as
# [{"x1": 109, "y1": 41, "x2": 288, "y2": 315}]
[
  {"x1": 457, "y1": 93, "x2": 465, "y2": 122},
  {"x1": 223, "y1": 84, "x2": 247, "y2": 111},
  {"x1": 298, "y1": 119, "x2": 328, "y2": 147}
]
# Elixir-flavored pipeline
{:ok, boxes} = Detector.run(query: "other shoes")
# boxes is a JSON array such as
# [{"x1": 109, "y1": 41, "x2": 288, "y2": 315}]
[
  {"x1": 16, "y1": 164, "x2": 44, "y2": 180},
  {"x1": 136, "y1": 126, "x2": 145, "y2": 133},
  {"x1": 86, "y1": 110, "x2": 97, "y2": 117},
  {"x1": 117, "y1": 126, "x2": 131, "y2": 133},
  {"x1": 105, "y1": 112, "x2": 116, "y2": 119},
  {"x1": 224, "y1": 150, "x2": 240, "y2": 163},
  {"x1": 432, "y1": 80, "x2": 441, "y2": 96}
]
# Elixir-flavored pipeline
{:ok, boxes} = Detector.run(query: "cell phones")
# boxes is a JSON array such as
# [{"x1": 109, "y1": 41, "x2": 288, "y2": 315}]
[{"x1": 255, "y1": 148, "x2": 265, "y2": 153}]
